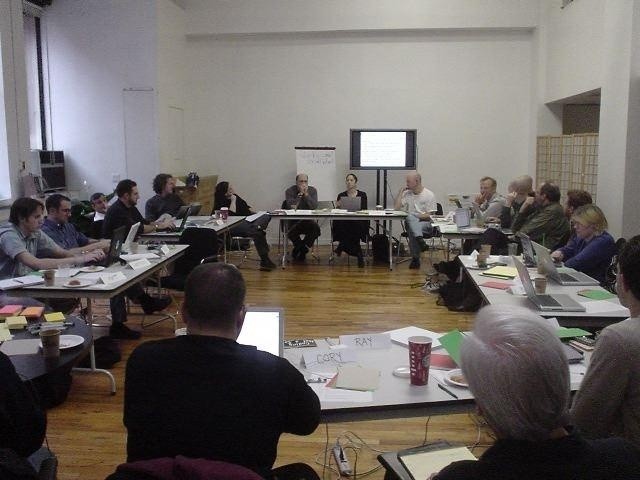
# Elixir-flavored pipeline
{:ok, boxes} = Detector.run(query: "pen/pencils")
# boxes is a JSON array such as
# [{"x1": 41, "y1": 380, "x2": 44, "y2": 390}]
[
  {"x1": 13, "y1": 278, "x2": 23, "y2": 284},
  {"x1": 25, "y1": 322, "x2": 74, "y2": 335},
  {"x1": 439, "y1": 385, "x2": 458, "y2": 399}
]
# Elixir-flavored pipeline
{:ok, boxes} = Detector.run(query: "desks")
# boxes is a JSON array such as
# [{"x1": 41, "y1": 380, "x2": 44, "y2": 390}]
[
  {"x1": 0, "y1": 214, "x2": 252, "y2": 394},
  {"x1": 282, "y1": 323, "x2": 599, "y2": 416},
  {"x1": 429, "y1": 212, "x2": 639, "y2": 337}
]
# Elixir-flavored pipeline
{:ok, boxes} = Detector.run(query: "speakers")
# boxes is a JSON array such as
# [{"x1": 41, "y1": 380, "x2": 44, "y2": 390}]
[{"x1": 24, "y1": 148, "x2": 67, "y2": 192}]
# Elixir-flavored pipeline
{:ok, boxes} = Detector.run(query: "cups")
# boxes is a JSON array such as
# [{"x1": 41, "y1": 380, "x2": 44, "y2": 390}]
[
  {"x1": 475, "y1": 244, "x2": 493, "y2": 268},
  {"x1": 376, "y1": 205, "x2": 383, "y2": 214},
  {"x1": 39, "y1": 329, "x2": 62, "y2": 360},
  {"x1": 506, "y1": 243, "x2": 518, "y2": 259},
  {"x1": 129, "y1": 241, "x2": 138, "y2": 254},
  {"x1": 119, "y1": 250, "x2": 129, "y2": 266},
  {"x1": 535, "y1": 277, "x2": 547, "y2": 294},
  {"x1": 408, "y1": 334, "x2": 432, "y2": 387},
  {"x1": 43, "y1": 270, "x2": 56, "y2": 287},
  {"x1": 221, "y1": 207, "x2": 229, "y2": 220},
  {"x1": 214, "y1": 210, "x2": 222, "y2": 220}
]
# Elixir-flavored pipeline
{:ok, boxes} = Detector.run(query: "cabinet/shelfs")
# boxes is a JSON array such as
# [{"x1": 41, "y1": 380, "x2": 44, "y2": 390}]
[{"x1": 535, "y1": 134, "x2": 599, "y2": 210}]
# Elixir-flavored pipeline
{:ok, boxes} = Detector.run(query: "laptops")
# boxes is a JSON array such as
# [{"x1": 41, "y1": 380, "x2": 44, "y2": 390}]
[
  {"x1": 519, "y1": 232, "x2": 536, "y2": 266},
  {"x1": 455, "y1": 198, "x2": 500, "y2": 234},
  {"x1": 511, "y1": 255, "x2": 587, "y2": 312},
  {"x1": 340, "y1": 197, "x2": 361, "y2": 210},
  {"x1": 530, "y1": 240, "x2": 601, "y2": 286},
  {"x1": 155, "y1": 207, "x2": 191, "y2": 232},
  {"x1": 235, "y1": 307, "x2": 284, "y2": 358},
  {"x1": 84, "y1": 225, "x2": 126, "y2": 267},
  {"x1": 122, "y1": 221, "x2": 141, "y2": 248},
  {"x1": 177, "y1": 204, "x2": 201, "y2": 219}
]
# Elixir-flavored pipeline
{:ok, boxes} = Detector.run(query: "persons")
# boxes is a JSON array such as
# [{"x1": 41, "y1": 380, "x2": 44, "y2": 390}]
[
  {"x1": 284, "y1": 175, "x2": 321, "y2": 261},
  {"x1": 394, "y1": 172, "x2": 437, "y2": 270},
  {"x1": 123, "y1": 263, "x2": 322, "y2": 478},
  {"x1": 569, "y1": 233, "x2": 639, "y2": 446},
  {"x1": 435, "y1": 172, "x2": 614, "y2": 286},
  {"x1": 332, "y1": 174, "x2": 369, "y2": 267},
  {"x1": 432, "y1": 302, "x2": 639, "y2": 478},
  {"x1": 212, "y1": 181, "x2": 278, "y2": 270}
]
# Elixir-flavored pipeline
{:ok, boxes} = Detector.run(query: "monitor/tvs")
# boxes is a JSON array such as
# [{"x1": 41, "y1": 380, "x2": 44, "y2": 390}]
[{"x1": 350, "y1": 128, "x2": 417, "y2": 171}]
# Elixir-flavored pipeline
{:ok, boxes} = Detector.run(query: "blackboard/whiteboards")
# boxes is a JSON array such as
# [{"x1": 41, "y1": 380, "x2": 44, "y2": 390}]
[{"x1": 295, "y1": 147, "x2": 337, "y2": 202}]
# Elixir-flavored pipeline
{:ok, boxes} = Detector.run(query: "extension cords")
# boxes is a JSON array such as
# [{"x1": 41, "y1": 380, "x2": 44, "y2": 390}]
[{"x1": 332, "y1": 445, "x2": 353, "y2": 476}]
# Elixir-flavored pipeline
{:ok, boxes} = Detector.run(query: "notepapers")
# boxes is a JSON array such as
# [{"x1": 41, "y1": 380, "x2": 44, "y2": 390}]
[
  {"x1": 0, "y1": 305, "x2": 23, "y2": 320},
  {"x1": 6, "y1": 316, "x2": 27, "y2": 333},
  {"x1": 18, "y1": 306, "x2": 45, "y2": 319},
  {"x1": 44, "y1": 311, "x2": 66, "y2": 322}
]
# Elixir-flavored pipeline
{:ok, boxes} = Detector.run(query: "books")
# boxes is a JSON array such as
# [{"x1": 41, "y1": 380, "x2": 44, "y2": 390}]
[
  {"x1": 382, "y1": 326, "x2": 446, "y2": 352},
  {"x1": 399, "y1": 446, "x2": 477, "y2": 480}
]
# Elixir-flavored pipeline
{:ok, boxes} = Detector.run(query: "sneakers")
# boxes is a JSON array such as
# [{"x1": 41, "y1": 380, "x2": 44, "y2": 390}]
[
  {"x1": 260, "y1": 256, "x2": 276, "y2": 270},
  {"x1": 291, "y1": 242, "x2": 309, "y2": 261}
]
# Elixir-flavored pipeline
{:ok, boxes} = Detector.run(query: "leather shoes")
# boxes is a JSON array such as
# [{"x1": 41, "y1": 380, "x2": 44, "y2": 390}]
[
  {"x1": 420, "y1": 242, "x2": 429, "y2": 252},
  {"x1": 109, "y1": 321, "x2": 143, "y2": 339},
  {"x1": 358, "y1": 258, "x2": 365, "y2": 267},
  {"x1": 332, "y1": 246, "x2": 342, "y2": 256},
  {"x1": 432, "y1": 258, "x2": 461, "y2": 283},
  {"x1": 409, "y1": 256, "x2": 420, "y2": 270},
  {"x1": 141, "y1": 295, "x2": 172, "y2": 315}
]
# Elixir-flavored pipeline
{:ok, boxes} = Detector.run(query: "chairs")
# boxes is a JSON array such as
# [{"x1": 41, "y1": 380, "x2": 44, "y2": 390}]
[{"x1": 271, "y1": 201, "x2": 445, "y2": 270}]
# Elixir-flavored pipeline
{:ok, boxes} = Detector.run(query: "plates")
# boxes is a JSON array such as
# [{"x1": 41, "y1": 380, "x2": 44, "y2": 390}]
[
  {"x1": 79, "y1": 265, "x2": 105, "y2": 273},
  {"x1": 445, "y1": 366, "x2": 470, "y2": 388},
  {"x1": 468, "y1": 228, "x2": 483, "y2": 233},
  {"x1": 63, "y1": 280, "x2": 93, "y2": 288},
  {"x1": 38, "y1": 334, "x2": 85, "y2": 350}
]
